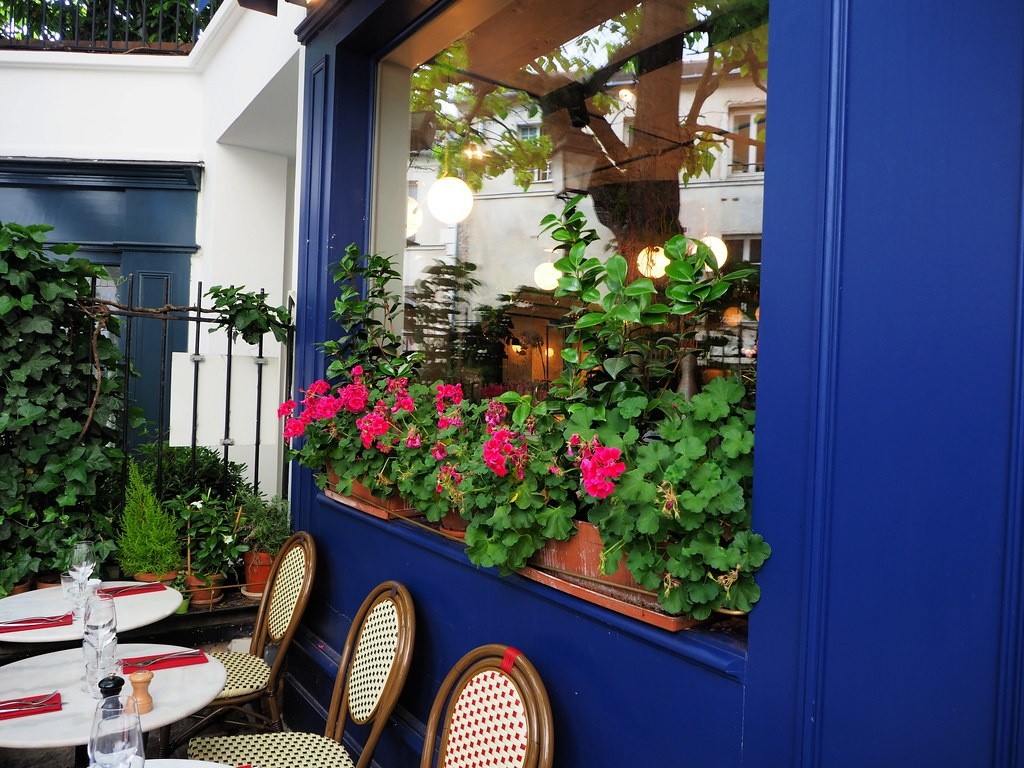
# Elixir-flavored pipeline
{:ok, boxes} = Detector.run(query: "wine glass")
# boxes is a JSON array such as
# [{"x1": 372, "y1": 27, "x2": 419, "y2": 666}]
[
  {"x1": 69, "y1": 546, "x2": 94, "y2": 606},
  {"x1": 83, "y1": 594, "x2": 118, "y2": 669},
  {"x1": 83, "y1": 602, "x2": 117, "y2": 659},
  {"x1": 74, "y1": 541, "x2": 97, "y2": 581}
]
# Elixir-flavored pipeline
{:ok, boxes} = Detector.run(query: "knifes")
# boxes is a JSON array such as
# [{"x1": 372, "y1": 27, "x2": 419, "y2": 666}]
[
  {"x1": 0, "y1": 617, "x2": 81, "y2": 626},
  {"x1": 0, "y1": 702, "x2": 68, "y2": 713}
]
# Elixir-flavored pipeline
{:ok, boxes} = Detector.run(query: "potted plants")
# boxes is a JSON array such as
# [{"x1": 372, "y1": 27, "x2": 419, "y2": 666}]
[
  {"x1": 0, "y1": 220, "x2": 143, "y2": 597},
  {"x1": 114, "y1": 458, "x2": 184, "y2": 586},
  {"x1": 167, "y1": 570, "x2": 191, "y2": 614},
  {"x1": 236, "y1": 486, "x2": 293, "y2": 592}
]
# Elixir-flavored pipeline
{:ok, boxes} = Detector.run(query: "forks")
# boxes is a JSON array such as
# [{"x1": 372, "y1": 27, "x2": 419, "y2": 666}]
[
  {"x1": 0, "y1": 611, "x2": 68, "y2": 621},
  {"x1": 0, "y1": 690, "x2": 58, "y2": 706}
]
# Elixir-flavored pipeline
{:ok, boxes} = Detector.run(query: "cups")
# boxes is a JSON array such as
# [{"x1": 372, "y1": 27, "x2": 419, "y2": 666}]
[
  {"x1": 86, "y1": 694, "x2": 145, "y2": 768},
  {"x1": 61, "y1": 571, "x2": 79, "y2": 597},
  {"x1": 94, "y1": 714, "x2": 140, "y2": 768},
  {"x1": 67, "y1": 586, "x2": 98, "y2": 621},
  {"x1": 85, "y1": 658, "x2": 124, "y2": 699}
]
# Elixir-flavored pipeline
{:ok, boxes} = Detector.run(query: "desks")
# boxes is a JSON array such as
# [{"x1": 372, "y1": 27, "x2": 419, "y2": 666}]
[
  {"x1": 0, "y1": 644, "x2": 227, "y2": 768},
  {"x1": 0, "y1": 582, "x2": 183, "y2": 644},
  {"x1": 145, "y1": 759, "x2": 236, "y2": 768}
]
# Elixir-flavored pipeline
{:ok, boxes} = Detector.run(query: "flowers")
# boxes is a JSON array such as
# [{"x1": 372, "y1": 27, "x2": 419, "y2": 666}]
[
  {"x1": 161, "y1": 486, "x2": 249, "y2": 588},
  {"x1": 273, "y1": 365, "x2": 773, "y2": 618}
]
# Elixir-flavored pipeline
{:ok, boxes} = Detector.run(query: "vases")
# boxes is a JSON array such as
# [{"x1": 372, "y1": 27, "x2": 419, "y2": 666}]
[
  {"x1": 527, "y1": 518, "x2": 677, "y2": 615},
  {"x1": 323, "y1": 461, "x2": 424, "y2": 517},
  {"x1": 184, "y1": 574, "x2": 226, "y2": 610},
  {"x1": 438, "y1": 506, "x2": 473, "y2": 535}
]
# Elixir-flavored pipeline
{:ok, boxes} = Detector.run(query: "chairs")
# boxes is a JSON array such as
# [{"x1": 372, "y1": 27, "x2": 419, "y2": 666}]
[
  {"x1": 144, "y1": 531, "x2": 315, "y2": 759},
  {"x1": 420, "y1": 643, "x2": 555, "y2": 768},
  {"x1": 187, "y1": 581, "x2": 415, "y2": 768}
]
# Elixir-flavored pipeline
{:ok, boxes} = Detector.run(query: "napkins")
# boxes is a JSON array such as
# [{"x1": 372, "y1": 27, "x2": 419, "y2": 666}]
[
  {"x1": 122, "y1": 648, "x2": 209, "y2": 674},
  {"x1": 0, "y1": 693, "x2": 63, "y2": 719},
  {"x1": 0, "y1": 614, "x2": 73, "y2": 633},
  {"x1": 97, "y1": 582, "x2": 167, "y2": 599}
]
